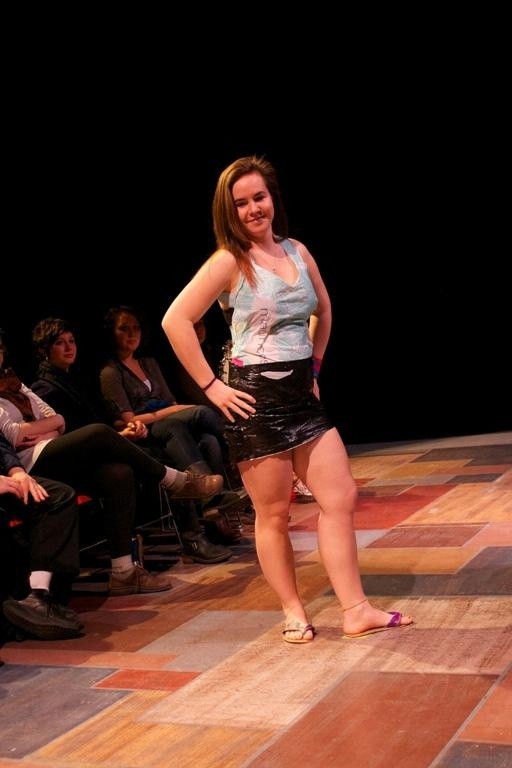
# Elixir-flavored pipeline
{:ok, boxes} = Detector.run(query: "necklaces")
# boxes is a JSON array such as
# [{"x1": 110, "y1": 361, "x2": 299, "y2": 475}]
[{"x1": 254, "y1": 243, "x2": 286, "y2": 273}]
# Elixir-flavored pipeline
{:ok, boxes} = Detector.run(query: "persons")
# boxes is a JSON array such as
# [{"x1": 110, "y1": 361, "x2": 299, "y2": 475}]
[{"x1": 161, "y1": 151, "x2": 412, "y2": 643}]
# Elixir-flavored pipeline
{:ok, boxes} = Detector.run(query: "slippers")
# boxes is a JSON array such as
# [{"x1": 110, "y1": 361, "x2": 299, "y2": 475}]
[
  {"x1": 282, "y1": 623, "x2": 315, "y2": 643},
  {"x1": 342, "y1": 611, "x2": 414, "y2": 638}
]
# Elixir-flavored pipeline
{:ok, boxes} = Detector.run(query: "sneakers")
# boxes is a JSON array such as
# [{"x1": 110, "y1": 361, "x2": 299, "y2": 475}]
[
  {"x1": 180, "y1": 532, "x2": 234, "y2": 564},
  {"x1": 2, "y1": 596, "x2": 83, "y2": 641},
  {"x1": 168, "y1": 470, "x2": 224, "y2": 502},
  {"x1": 107, "y1": 565, "x2": 172, "y2": 596},
  {"x1": 205, "y1": 517, "x2": 241, "y2": 545}
]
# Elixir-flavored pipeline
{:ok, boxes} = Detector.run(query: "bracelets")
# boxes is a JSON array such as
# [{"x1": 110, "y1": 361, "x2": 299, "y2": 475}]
[{"x1": 311, "y1": 357, "x2": 322, "y2": 377}]
